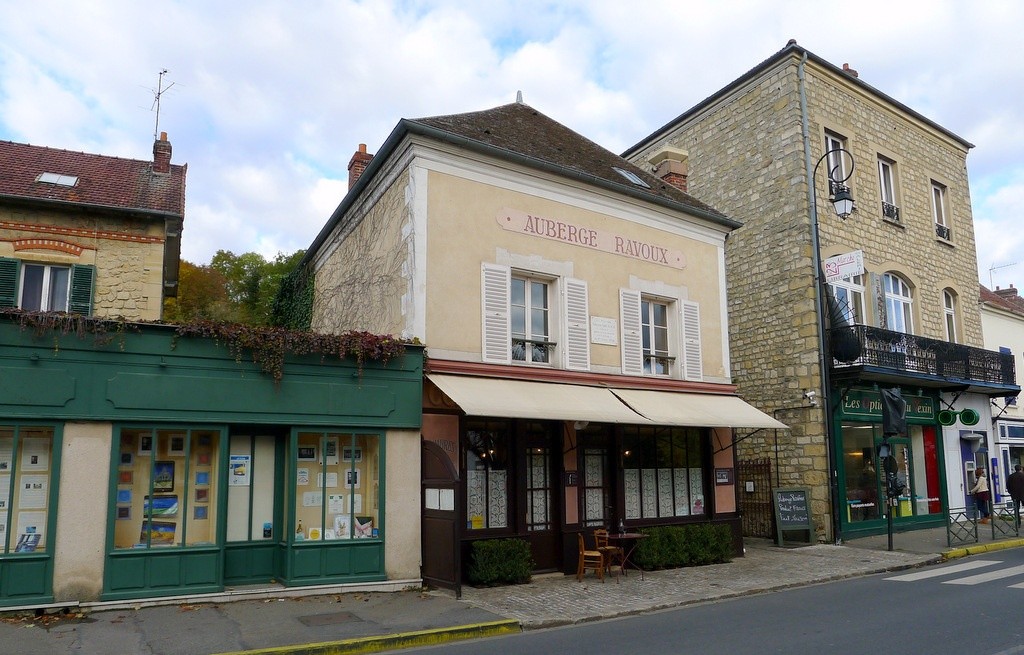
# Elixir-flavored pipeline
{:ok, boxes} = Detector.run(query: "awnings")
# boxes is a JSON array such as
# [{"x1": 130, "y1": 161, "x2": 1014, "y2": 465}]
[{"x1": 425, "y1": 372, "x2": 791, "y2": 430}]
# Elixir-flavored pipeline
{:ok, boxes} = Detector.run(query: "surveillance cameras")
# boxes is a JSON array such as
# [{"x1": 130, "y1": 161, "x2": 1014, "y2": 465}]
[{"x1": 805, "y1": 391, "x2": 815, "y2": 398}]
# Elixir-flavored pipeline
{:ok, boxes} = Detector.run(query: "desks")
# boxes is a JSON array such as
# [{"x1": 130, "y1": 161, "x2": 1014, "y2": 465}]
[{"x1": 589, "y1": 533, "x2": 649, "y2": 571}]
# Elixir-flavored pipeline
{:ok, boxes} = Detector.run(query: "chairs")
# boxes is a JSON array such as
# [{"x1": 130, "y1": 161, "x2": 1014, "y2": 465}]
[
  {"x1": 593, "y1": 530, "x2": 624, "y2": 575},
  {"x1": 576, "y1": 533, "x2": 604, "y2": 584}
]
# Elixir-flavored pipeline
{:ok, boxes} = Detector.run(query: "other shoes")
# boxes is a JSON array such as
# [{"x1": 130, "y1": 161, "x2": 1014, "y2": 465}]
[{"x1": 977, "y1": 519, "x2": 987, "y2": 522}]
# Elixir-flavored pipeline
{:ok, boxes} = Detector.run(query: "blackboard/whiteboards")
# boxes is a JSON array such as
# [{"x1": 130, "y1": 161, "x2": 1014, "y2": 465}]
[{"x1": 771, "y1": 487, "x2": 814, "y2": 531}]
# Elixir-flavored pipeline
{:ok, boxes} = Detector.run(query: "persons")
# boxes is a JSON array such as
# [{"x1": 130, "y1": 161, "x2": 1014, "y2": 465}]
[
  {"x1": 969, "y1": 468, "x2": 992, "y2": 524},
  {"x1": 1006, "y1": 466, "x2": 1024, "y2": 528}
]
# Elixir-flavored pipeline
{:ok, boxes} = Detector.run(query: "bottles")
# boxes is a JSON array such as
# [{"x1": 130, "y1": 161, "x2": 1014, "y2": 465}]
[
  {"x1": 296, "y1": 519, "x2": 303, "y2": 534},
  {"x1": 618, "y1": 518, "x2": 624, "y2": 534}
]
